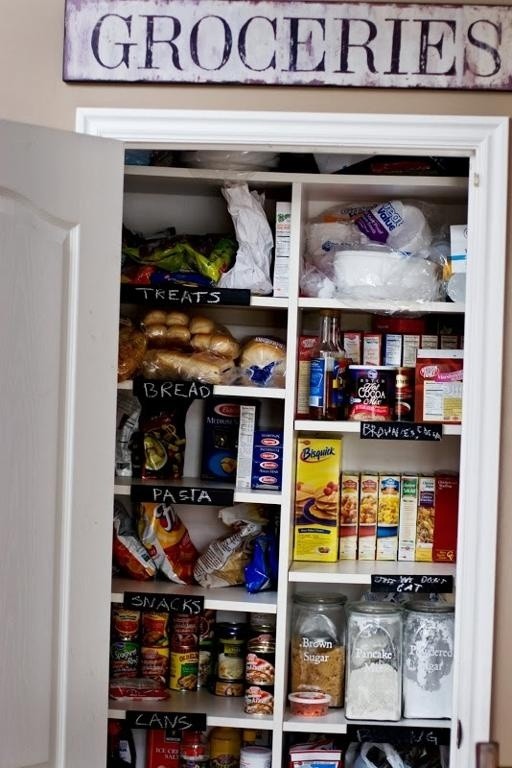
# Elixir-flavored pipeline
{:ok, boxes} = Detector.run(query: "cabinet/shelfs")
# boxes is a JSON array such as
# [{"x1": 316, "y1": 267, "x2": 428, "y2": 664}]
[{"x1": 0, "y1": 106, "x2": 509, "y2": 767}]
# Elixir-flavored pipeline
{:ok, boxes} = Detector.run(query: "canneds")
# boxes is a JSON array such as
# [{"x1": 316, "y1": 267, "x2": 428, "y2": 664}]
[
  {"x1": 290, "y1": 591, "x2": 456, "y2": 722},
  {"x1": 332, "y1": 355, "x2": 414, "y2": 423},
  {"x1": 111, "y1": 605, "x2": 275, "y2": 717},
  {"x1": 240, "y1": 745, "x2": 271, "y2": 767}
]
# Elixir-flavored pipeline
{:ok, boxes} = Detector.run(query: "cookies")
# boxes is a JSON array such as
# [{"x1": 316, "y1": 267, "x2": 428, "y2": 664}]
[{"x1": 295, "y1": 477, "x2": 337, "y2": 524}]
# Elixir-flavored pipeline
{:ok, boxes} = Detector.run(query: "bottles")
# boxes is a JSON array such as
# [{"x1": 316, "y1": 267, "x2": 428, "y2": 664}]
[
  {"x1": 402, "y1": 600, "x2": 454, "y2": 719},
  {"x1": 290, "y1": 590, "x2": 348, "y2": 708},
  {"x1": 344, "y1": 600, "x2": 403, "y2": 721},
  {"x1": 308, "y1": 308, "x2": 347, "y2": 421}
]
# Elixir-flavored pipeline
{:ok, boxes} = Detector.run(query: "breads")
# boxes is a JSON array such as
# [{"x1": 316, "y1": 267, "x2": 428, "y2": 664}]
[{"x1": 118, "y1": 308, "x2": 284, "y2": 394}]
